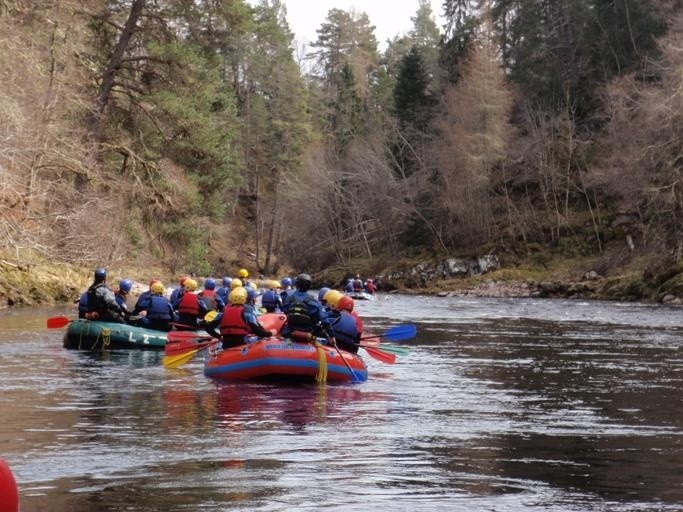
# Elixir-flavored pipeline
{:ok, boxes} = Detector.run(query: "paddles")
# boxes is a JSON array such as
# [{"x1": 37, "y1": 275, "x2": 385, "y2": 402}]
[
  {"x1": 47, "y1": 318, "x2": 88, "y2": 328},
  {"x1": 322, "y1": 329, "x2": 365, "y2": 384},
  {"x1": 352, "y1": 341, "x2": 408, "y2": 365},
  {"x1": 204, "y1": 311, "x2": 219, "y2": 322},
  {"x1": 360, "y1": 324, "x2": 417, "y2": 341},
  {"x1": 163, "y1": 332, "x2": 223, "y2": 368}
]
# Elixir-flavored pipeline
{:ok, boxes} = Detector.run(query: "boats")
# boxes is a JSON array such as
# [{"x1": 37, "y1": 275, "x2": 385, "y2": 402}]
[
  {"x1": 255, "y1": 311, "x2": 286, "y2": 335},
  {"x1": 201, "y1": 332, "x2": 370, "y2": 386},
  {"x1": 60, "y1": 316, "x2": 220, "y2": 359},
  {"x1": 0, "y1": 455, "x2": 19, "y2": 511},
  {"x1": 343, "y1": 290, "x2": 377, "y2": 303}
]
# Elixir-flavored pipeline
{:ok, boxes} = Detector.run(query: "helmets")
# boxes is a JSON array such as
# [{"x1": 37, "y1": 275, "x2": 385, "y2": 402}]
[
  {"x1": 229, "y1": 287, "x2": 247, "y2": 304},
  {"x1": 95, "y1": 268, "x2": 106, "y2": 277},
  {"x1": 183, "y1": 279, "x2": 197, "y2": 290},
  {"x1": 205, "y1": 278, "x2": 216, "y2": 289},
  {"x1": 150, "y1": 280, "x2": 161, "y2": 287},
  {"x1": 348, "y1": 274, "x2": 372, "y2": 283},
  {"x1": 269, "y1": 273, "x2": 312, "y2": 289},
  {"x1": 318, "y1": 287, "x2": 354, "y2": 312},
  {"x1": 120, "y1": 279, "x2": 131, "y2": 292},
  {"x1": 223, "y1": 269, "x2": 248, "y2": 290},
  {"x1": 181, "y1": 277, "x2": 189, "y2": 285},
  {"x1": 151, "y1": 283, "x2": 164, "y2": 295}
]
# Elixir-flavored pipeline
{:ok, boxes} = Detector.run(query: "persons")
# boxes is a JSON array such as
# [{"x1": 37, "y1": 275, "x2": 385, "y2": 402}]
[{"x1": 79, "y1": 268, "x2": 378, "y2": 353}]
